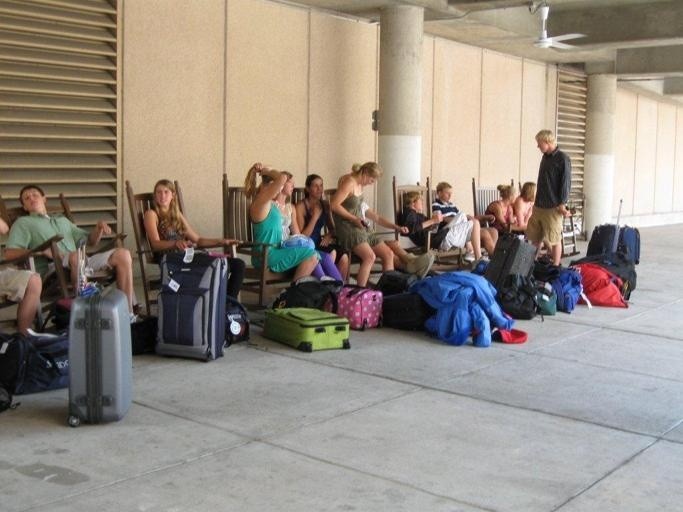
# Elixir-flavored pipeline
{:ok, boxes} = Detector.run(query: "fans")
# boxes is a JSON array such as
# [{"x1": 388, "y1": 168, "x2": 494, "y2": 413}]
[{"x1": 480, "y1": 1, "x2": 588, "y2": 49}]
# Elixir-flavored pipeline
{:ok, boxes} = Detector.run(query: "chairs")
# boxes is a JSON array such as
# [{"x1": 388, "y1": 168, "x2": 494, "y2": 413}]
[{"x1": 0, "y1": 174, "x2": 583, "y2": 332}]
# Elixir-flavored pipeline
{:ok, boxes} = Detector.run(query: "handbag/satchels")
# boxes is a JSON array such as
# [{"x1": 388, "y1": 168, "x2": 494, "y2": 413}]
[
  {"x1": 16, "y1": 328, "x2": 69, "y2": 393},
  {"x1": 337, "y1": 284, "x2": 384, "y2": 330},
  {"x1": 375, "y1": 270, "x2": 417, "y2": 293}
]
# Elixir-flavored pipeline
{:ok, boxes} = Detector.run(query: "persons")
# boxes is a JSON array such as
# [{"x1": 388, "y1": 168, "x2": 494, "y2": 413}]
[
  {"x1": 275, "y1": 168, "x2": 346, "y2": 285},
  {"x1": 522, "y1": 128, "x2": 572, "y2": 268},
  {"x1": 483, "y1": 182, "x2": 528, "y2": 236},
  {"x1": 360, "y1": 201, "x2": 436, "y2": 281},
  {"x1": 398, "y1": 189, "x2": 489, "y2": 263},
  {"x1": 240, "y1": 161, "x2": 322, "y2": 281},
  {"x1": 329, "y1": 158, "x2": 395, "y2": 288},
  {"x1": 430, "y1": 180, "x2": 499, "y2": 259},
  {"x1": 142, "y1": 178, "x2": 245, "y2": 308},
  {"x1": 513, "y1": 180, "x2": 552, "y2": 255},
  {"x1": 4, "y1": 183, "x2": 146, "y2": 325},
  {"x1": 294, "y1": 171, "x2": 350, "y2": 285},
  {"x1": 0, "y1": 198, "x2": 44, "y2": 338}
]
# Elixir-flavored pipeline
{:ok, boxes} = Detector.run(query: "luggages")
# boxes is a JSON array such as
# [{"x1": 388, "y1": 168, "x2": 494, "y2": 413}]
[
  {"x1": 68, "y1": 236, "x2": 132, "y2": 428},
  {"x1": 381, "y1": 291, "x2": 432, "y2": 329},
  {"x1": 262, "y1": 305, "x2": 351, "y2": 353},
  {"x1": 484, "y1": 229, "x2": 538, "y2": 291},
  {"x1": 155, "y1": 249, "x2": 229, "y2": 361},
  {"x1": 586, "y1": 198, "x2": 624, "y2": 255}
]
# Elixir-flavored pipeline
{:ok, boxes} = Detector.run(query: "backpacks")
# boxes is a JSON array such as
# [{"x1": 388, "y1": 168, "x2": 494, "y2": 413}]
[
  {"x1": 494, "y1": 255, "x2": 592, "y2": 321},
  {"x1": 618, "y1": 225, "x2": 639, "y2": 264},
  {"x1": 226, "y1": 297, "x2": 250, "y2": 343},
  {"x1": 0, "y1": 331, "x2": 28, "y2": 410},
  {"x1": 272, "y1": 280, "x2": 338, "y2": 312}
]
